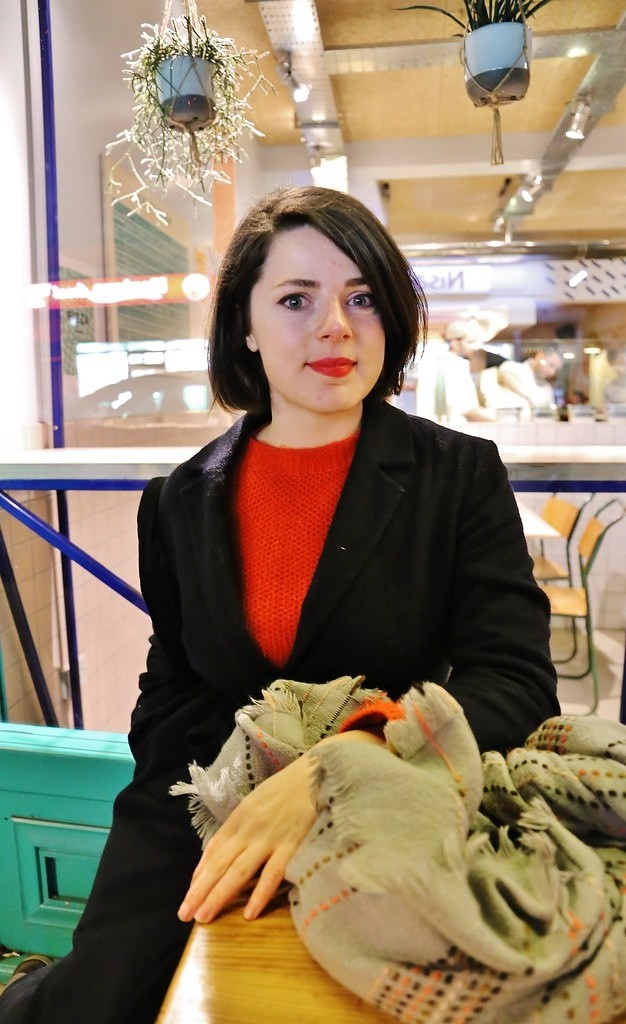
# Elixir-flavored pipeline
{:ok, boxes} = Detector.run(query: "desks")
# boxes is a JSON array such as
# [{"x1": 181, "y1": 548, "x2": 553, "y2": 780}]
[
  {"x1": 155, "y1": 881, "x2": 394, "y2": 1024},
  {"x1": 517, "y1": 500, "x2": 561, "y2": 539},
  {"x1": 0, "y1": 447, "x2": 626, "y2": 732}
]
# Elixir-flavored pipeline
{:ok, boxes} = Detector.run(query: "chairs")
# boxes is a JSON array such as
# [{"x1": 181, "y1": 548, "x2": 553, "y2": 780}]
[
  {"x1": 531, "y1": 492, "x2": 595, "y2": 671},
  {"x1": 538, "y1": 498, "x2": 626, "y2": 717}
]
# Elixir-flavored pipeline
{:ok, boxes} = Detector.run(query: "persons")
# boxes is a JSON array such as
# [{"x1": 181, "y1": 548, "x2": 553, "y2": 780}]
[
  {"x1": 442, "y1": 318, "x2": 508, "y2": 408},
  {"x1": 480, "y1": 344, "x2": 565, "y2": 423},
  {"x1": 0, "y1": 189, "x2": 564, "y2": 1024}
]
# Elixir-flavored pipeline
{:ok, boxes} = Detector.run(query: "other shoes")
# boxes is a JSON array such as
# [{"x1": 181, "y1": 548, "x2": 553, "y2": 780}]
[{"x1": 12, "y1": 956, "x2": 56, "y2": 975}]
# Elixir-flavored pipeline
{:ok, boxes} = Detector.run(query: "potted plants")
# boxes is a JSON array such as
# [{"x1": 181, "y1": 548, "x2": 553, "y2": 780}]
[
  {"x1": 392, "y1": 0, "x2": 552, "y2": 108},
  {"x1": 104, "y1": 14, "x2": 277, "y2": 226}
]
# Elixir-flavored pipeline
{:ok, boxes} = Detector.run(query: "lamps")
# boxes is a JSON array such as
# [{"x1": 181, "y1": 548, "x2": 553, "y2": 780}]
[
  {"x1": 521, "y1": 181, "x2": 545, "y2": 203},
  {"x1": 563, "y1": 101, "x2": 591, "y2": 139},
  {"x1": 275, "y1": 52, "x2": 309, "y2": 103}
]
